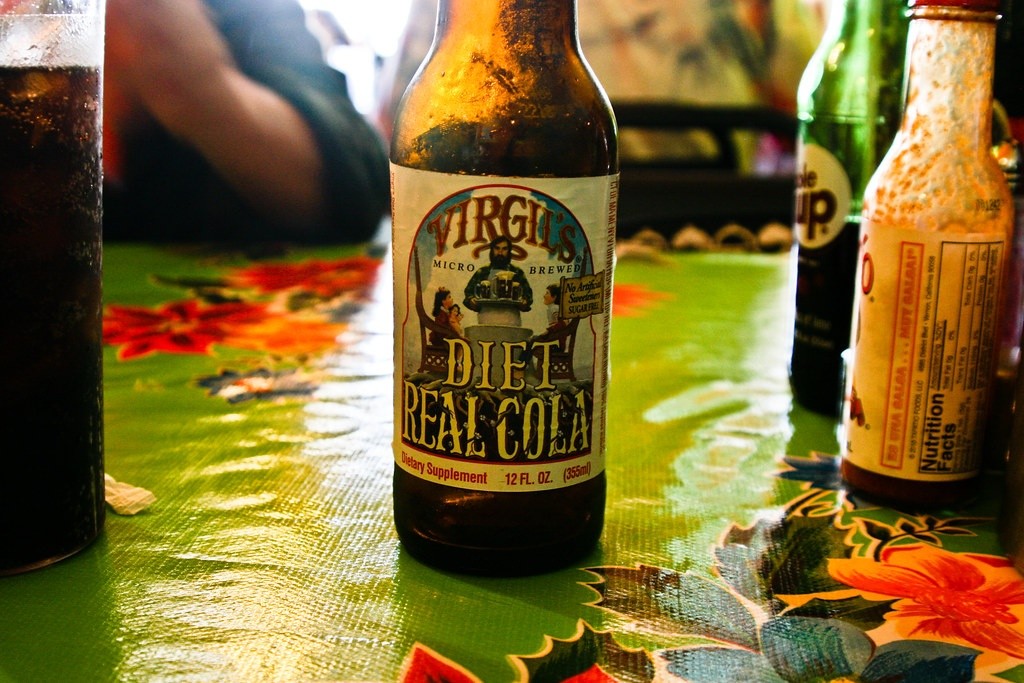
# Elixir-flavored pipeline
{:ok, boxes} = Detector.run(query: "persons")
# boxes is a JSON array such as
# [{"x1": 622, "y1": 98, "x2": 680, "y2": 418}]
[{"x1": 98, "y1": 0, "x2": 392, "y2": 248}]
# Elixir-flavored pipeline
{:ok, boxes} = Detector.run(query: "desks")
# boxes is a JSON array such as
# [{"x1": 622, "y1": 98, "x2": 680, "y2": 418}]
[{"x1": 1, "y1": 236, "x2": 1024, "y2": 683}]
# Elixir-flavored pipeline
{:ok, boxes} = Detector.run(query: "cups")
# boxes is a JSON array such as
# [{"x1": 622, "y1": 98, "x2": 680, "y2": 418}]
[{"x1": 0, "y1": 0, "x2": 106, "y2": 573}]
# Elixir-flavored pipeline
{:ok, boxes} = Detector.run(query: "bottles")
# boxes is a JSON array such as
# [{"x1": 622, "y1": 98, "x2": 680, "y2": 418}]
[
  {"x1": 841, "y1": 0, "x2": 1015, "y2": 508},
  {"x1": 388, "y1": 0, "x2": 620, "y2": 580},
  {"x1": 789, "y1": 0, "x2": 911, "y2": 418}
]
